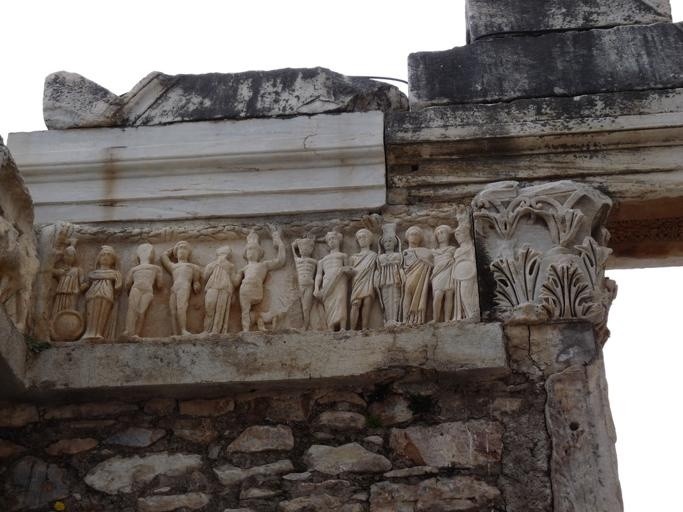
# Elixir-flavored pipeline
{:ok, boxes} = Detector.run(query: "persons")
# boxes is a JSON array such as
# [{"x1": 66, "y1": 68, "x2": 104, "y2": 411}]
[
  {"x1": 292, "y1": 225, "x2": 478, "y2": 332},
  {"x1": 162, "y1": 231, "x2": 286, "y2": 337},
  {"x1": 50, "y1": 243, "x2": 163, "y2": 341}
]
